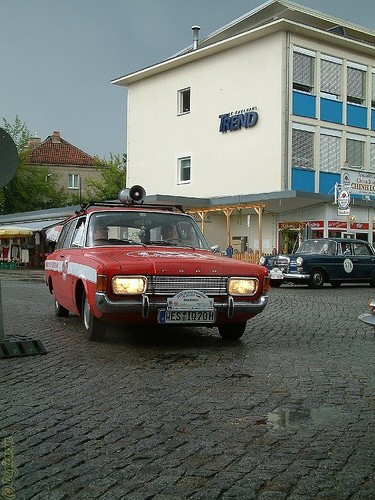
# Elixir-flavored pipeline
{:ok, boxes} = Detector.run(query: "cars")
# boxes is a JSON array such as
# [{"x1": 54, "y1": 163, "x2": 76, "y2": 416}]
[
  {"x1": 258, "y1": 236, "x2": 375, "y2": 289},
  {"x1": 44, "y1": 185, "x2": 271, "y2": 343}
]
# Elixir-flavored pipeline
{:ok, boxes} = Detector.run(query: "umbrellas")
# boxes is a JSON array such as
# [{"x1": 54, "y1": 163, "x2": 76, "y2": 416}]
[{"x1": 0, "y1": 227, "x2": 33, "y2": 259}]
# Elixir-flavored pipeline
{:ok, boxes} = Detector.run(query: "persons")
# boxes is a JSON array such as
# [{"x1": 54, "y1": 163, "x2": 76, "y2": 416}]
[
  {"x1": 160, "y1": 223, "x2": 176, "y2": 242},
  {"x1": 91, "y1": 219, "x2": 110, "y2": 240}
]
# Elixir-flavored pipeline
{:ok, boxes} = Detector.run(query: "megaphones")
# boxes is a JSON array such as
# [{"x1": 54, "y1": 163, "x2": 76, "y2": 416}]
[{"x1": 118, "y1": 184, "x2": 145, "y2": 204}]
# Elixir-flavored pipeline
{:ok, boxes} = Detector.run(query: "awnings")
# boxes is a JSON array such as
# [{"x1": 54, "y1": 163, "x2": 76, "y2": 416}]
[{"x1": 0, "y1": 218, "x2": 67, "y2": 231}]
